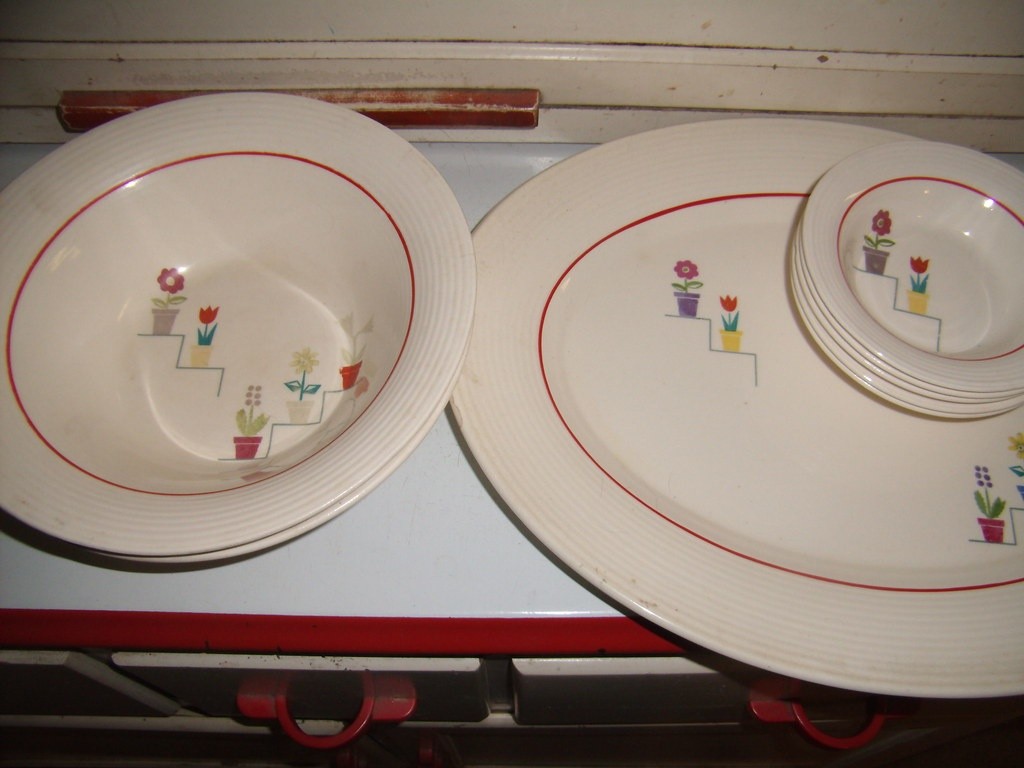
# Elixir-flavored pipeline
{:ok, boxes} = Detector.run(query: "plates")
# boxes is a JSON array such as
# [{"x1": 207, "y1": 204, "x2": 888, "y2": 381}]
[
  {"x1": 0, "y1": 93, "x2": 476, "y2": 564},
  {"x1": 789, "y1": 140, "x2": 1024, "y2": 419},
  {"x1": 451, "y1": 118, "x2": 1024, "y2": 698}
]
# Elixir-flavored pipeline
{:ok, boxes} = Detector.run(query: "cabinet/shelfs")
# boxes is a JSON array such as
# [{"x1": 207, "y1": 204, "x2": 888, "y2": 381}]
[{"x1": 0, "y1": 3, "x2": 1023, "y2": 767}]
